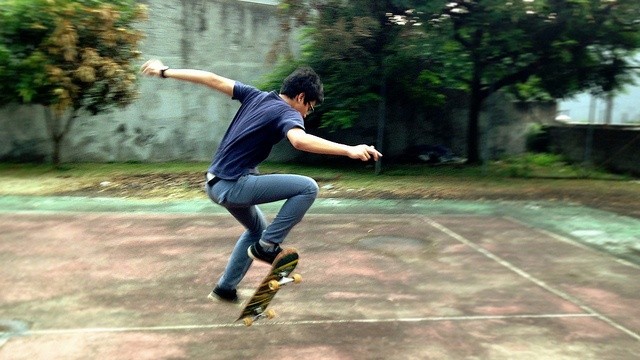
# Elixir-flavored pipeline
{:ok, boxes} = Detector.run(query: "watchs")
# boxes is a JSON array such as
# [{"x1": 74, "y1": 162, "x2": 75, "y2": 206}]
[{"x1": 160, "y1": 65, "x2": 169, "y2": 79}]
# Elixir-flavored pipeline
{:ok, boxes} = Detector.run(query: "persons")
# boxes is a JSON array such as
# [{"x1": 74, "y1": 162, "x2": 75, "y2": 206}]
[{"x1": 140, "y1": 56, "x2": 383, "y2": 306}]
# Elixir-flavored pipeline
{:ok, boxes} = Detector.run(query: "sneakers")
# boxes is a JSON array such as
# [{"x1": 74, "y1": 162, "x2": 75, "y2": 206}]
[
  {"x1": 207, "y1": 286, "x2": 242, "y2": 309},
  {"x1": 247, "y1": 241, "x2": 283, "y2": 266}
]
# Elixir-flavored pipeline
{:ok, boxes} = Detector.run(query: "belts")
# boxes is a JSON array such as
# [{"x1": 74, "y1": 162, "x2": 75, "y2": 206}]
[{"x1": 205, "y1": 172, "x2": 220, "y2": 188}]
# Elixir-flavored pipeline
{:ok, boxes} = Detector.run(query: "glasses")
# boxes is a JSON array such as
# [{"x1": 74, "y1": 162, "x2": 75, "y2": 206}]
[{"x1": 307, "y1": 98, "x2": 315, "y2": 114}]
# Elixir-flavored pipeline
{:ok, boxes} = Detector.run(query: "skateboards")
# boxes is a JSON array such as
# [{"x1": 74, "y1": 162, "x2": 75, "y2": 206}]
[{"x1": 230, "y1": 246, "x2": 303, "y2": 327}]
